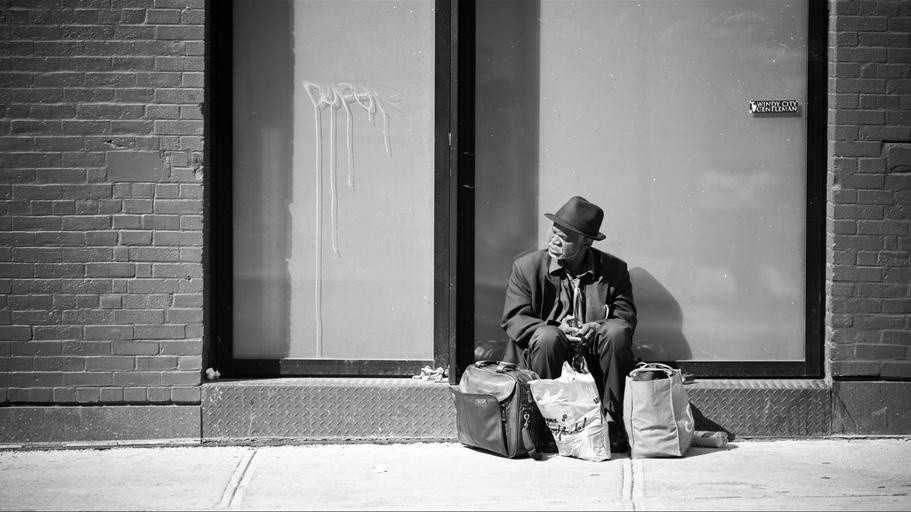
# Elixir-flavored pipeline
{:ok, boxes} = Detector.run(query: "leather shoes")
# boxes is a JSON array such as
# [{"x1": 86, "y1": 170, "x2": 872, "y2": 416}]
[
  {"x1": 612, "y1": 427, "x2": 628, "y2": 452},
  {"x1": 542, "y1": 438, "x2": 557, "y2": 453}
]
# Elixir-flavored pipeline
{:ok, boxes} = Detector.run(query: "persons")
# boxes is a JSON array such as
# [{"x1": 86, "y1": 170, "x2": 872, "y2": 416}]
[{"x1": 500, "y1": 196, "x2": 638, "y2": 453}]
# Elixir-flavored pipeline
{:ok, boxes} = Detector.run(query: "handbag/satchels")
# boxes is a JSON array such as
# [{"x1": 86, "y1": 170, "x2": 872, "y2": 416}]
[
  {"x1": 623, "y1": 362, "x2": 696, "y2": 456},
  {"x1": 451, "y1": 360, "x2": 539, "y2": 458}
]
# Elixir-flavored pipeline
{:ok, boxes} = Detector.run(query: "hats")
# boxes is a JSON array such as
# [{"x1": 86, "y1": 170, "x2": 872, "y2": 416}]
[{"x1": 545, "y1": 197, "x2": 605, "y2": 240}]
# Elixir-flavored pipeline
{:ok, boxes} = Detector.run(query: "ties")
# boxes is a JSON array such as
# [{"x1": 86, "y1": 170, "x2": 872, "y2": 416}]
[{"x1": 571, "y1": 279, "x2": 583, "y2": 325}]
